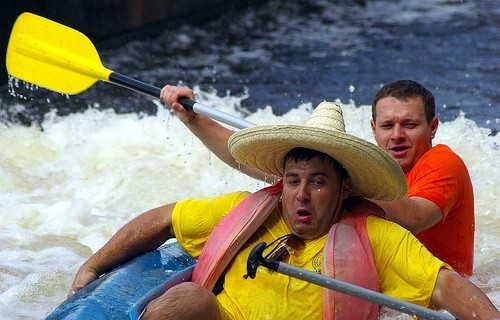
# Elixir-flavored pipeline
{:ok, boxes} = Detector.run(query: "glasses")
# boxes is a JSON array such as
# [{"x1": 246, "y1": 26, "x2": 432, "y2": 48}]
[{"x1": 264, "y1": 234, "x2": 307, "y2": 270}]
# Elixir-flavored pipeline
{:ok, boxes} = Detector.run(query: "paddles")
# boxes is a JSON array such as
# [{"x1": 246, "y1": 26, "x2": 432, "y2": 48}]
[
  {"x1": 5, "y1": 12, "x2": 257, "y2": 129},
  {"x1": 247, "y1": 241, "x2": 455, "y2": 320}
]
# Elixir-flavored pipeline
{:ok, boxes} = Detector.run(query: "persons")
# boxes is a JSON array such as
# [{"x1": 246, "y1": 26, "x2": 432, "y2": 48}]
[
  {"x1": 66, "y1": 100, "x2": 500, "y2": 319},
  {"x1": 158, "y1": 80, "x2": 476, "y2": 279}
]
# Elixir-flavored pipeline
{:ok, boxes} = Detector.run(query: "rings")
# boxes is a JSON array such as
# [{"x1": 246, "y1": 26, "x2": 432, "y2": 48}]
[{"x1": 71, "y1": 290, "x2": 76, "y2": 294}]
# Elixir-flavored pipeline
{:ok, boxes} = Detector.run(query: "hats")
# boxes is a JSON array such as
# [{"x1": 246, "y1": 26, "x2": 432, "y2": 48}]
[{"x1": 227, "y1": 99, "x2": 408, "y2": 202}]
